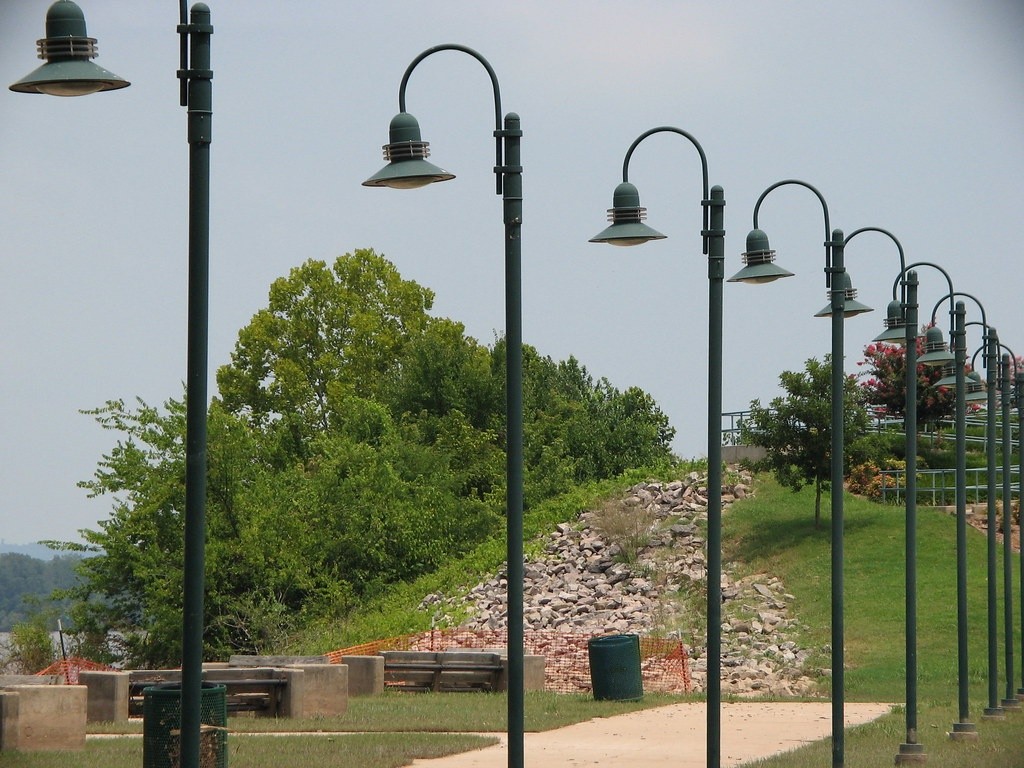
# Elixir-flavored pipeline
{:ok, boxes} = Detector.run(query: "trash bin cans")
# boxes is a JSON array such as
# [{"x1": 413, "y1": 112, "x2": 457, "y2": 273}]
[
  {"x1": 142, "y1": 681, "x2": 227, "y2": 768},
  {"x1": 587, "y1": 634, "x2": 645, "y2": 701}
]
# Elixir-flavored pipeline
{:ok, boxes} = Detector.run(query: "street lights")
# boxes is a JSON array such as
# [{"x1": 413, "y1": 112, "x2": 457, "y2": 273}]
[
  {"x1": 6, "y1": 1, "x2": 212, "y2": 768},
  {"x1": 930, "y1": 322, "x2": 1021, "y2": 712},
  {"x1": 914, "y1": 291, "x2": 1003, "y2": 717},
  {"x1": 964, "y1": 342, "x2": 1024, "y2": 700},
  {"x1": 928, "y1": 296, "x2": 1012, "y2": 724},
  {"x1": 724, "y1": 178, "x2": 851, "y2": 768},
  {"x1": 588, "y1": 121, "x2": 727, "y2": 768},
  {"x1": 360, "y1": 42, "x2": 525, "y2": 768},
  {"x1": 813, "y1": 223, "x2": 930, "y2": 768},
  {"x1": 872, "y1": 259, "x2": 979, "y2": 745}
]
